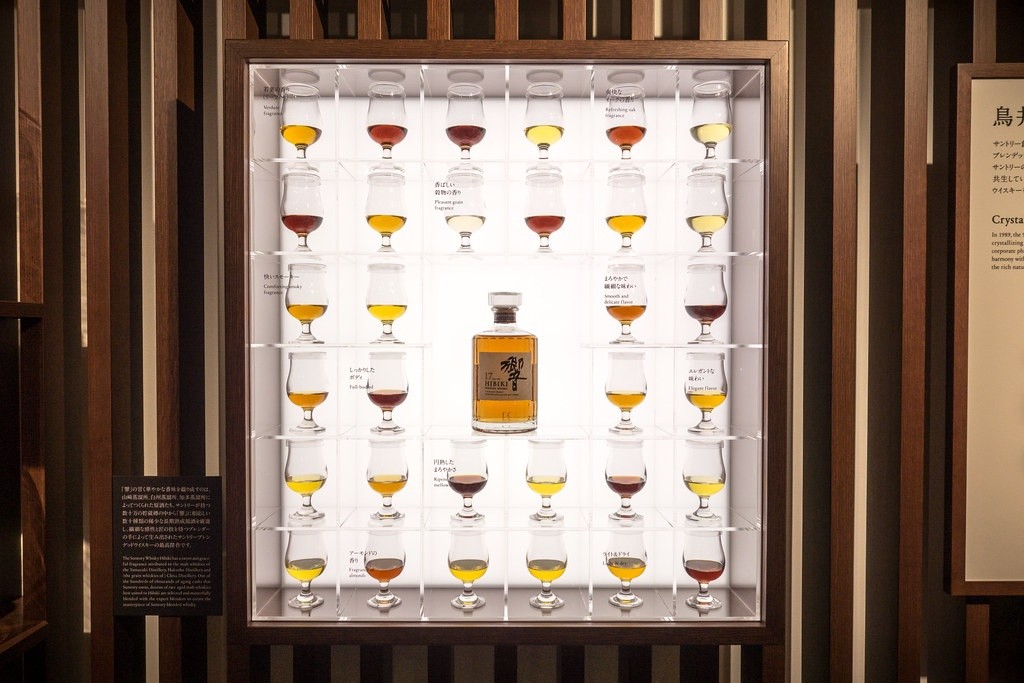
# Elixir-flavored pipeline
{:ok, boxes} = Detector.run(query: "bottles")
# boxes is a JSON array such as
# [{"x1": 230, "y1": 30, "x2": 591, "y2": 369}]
[{"x1": 472, "y1": 292, "x2": 538, "y2": 435}]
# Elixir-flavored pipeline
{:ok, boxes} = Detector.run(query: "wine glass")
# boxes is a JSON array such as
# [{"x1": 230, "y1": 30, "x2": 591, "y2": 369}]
[
  {"x1": 525, "y1": 85, "x2": 564, "y2": 158},
  {"x1": 367, "y1": 263, "x2": 407, "y2": 343},
  {"x1": 367, "y1": 84, "x2": 408, "y2": 160},
  {"x1": 446, "y1": 85, "x2": 486, "y2": 158},
  {"x1": 684, "y1": 264, "x2": 727, "y2": 344},
  {"x1": 525, "y1": 171, "x2": 565, "y2": 248},
  {"x1": 686, "y1": 173, "x2": 729, "y2": 252},
  {"x1": 285, "y1": 264, "x2": 328, "y2": 344},
  {"x1": 605, "y1": 85, "x2": 647, "y2": 159},
  {"x1": 285, "y1": 348, "x2": 728, "y2": 609},
  {"x1": 605, "y1": 173, "x2": 647, "y2": 250},
  {"x1": 606, "y1": 264, "x2": 647, "y2": 345},
  {"x1": 366, "y1": 352, "x2": 408, "y2": 434},
  {"x1": 280, "y1": 85, "x2": 322, "y2": 157},
  {"x1": 366, "y1": 173, "x2": 407, "y2": 252},
  {"x1": 286, "y1": 353, "x2": 329, "y2": 432},
  {"x1": 445, "y1": 175, "x2": 486, "y2": 251},
  {"x1": 280, "y1": 174, "x2": 322, "y2": 251},
  {"x1": 689, "y1": 83, "x2": 733, "y2": 159}
]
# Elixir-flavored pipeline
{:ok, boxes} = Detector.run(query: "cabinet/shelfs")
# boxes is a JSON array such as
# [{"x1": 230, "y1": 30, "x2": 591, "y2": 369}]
[{"x1": 219, "y1": 37, "x2": 796, "y2": 650}]
